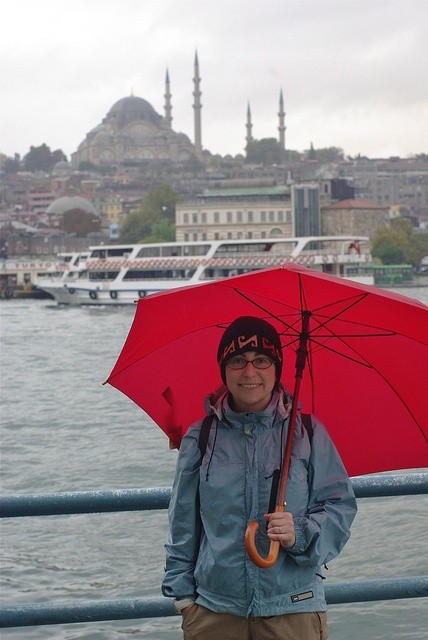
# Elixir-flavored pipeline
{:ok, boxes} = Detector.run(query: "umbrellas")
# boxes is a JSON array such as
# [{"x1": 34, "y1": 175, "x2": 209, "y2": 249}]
[{"x1": 102, "y1": 258, "x2": 428, "y2": 570}]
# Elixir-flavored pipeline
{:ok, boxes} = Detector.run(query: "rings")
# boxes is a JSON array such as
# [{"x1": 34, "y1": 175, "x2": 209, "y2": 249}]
[{"x1": 278, "y1": 526, "x2": 282, "y2": 534}]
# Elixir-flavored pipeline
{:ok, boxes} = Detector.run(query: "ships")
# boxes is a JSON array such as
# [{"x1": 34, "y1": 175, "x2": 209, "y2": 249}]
[{"x1": 28, "y1": 235, "x2": 376, "y2": 303}]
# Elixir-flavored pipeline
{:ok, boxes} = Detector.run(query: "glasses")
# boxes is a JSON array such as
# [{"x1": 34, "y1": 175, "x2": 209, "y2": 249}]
[{"x1": 225, "y1": 355, "x2": 276, "y2": 370}]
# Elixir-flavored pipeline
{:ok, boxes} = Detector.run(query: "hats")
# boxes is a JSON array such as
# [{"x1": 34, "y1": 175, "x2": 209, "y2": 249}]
[{"x1": 217, "y1": 316, "x2": 283, "y2": 386}]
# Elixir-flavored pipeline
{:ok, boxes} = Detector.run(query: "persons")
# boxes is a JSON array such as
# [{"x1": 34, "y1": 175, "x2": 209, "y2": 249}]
[{"x1": 160, "y1": 313, "x2": 360, "y2": 640}]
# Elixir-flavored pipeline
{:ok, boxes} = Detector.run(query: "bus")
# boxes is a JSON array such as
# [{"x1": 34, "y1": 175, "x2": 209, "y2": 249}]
[{"x1": 345, "y1": 265, "x2": 413, "y2": 284}]
